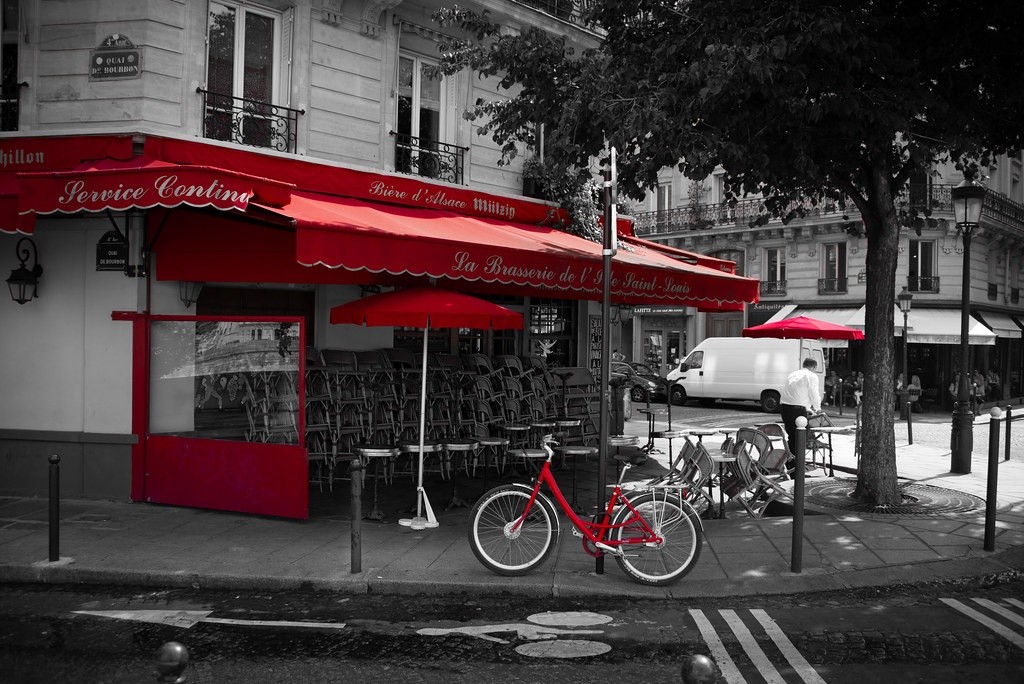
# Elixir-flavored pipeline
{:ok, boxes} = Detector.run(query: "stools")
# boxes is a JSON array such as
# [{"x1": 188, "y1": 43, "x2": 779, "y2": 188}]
[
  {"x1": 438, "y1": 438, "x2": 479, "y2": 511},
  {"x1": 398, "y1": 440, "x2": 443, "y2": 515},
  {"x1": 478, "y1": 437, "x2": 510, "y2": 487},
  {"x1": 351, "y1": 444, "x2": 402, "y2": 523},
  {"x1": 497, "y1": 418, "x2": 737, "y2": 515}
]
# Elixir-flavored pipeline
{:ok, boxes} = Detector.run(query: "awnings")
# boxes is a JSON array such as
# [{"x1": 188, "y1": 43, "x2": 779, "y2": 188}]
[{"x1": 0, "y1": 128, "x2": 1024, "y2": 344}]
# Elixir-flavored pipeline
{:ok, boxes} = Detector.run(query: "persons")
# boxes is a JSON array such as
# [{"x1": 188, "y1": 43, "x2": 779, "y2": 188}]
[
  {"x1": 822, "y1": 369, "x2": 999, "y2": 416},
  {"x1": 780, "y1": 359, "x2": 822, "y2": 479}
]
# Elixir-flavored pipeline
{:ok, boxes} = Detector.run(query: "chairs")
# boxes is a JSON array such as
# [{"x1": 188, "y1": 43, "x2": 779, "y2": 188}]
[
  {"x1": 646, "y1": 424, "x2": 829, "y2": 518},
  {"x1": 244, "y1": 348, "x2": 614, "y2": 490}
]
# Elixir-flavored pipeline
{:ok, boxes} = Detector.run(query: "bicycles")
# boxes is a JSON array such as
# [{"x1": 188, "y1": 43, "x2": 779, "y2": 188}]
[{"x1": 466, "y1": 428, "x2": 705, "y2": 586}]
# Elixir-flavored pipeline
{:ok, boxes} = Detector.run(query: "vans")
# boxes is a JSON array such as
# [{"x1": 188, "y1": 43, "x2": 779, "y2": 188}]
[{"x1": 665, "y1": 335, "x2": 827, "y2": 413}]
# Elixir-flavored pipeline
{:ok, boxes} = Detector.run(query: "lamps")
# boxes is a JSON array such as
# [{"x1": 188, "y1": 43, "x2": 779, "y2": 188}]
[{"x1": 5, "y1": 237, "x2": 43, "y2": 305}]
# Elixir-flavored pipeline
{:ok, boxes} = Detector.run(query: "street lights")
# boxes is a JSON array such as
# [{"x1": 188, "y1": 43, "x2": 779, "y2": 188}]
[
  {"x1": 949, "y1": 176, "x2": 987, "y2": 475},
  {"x1": 897, "y1": 285, "x2": 912, "y2": 420}
]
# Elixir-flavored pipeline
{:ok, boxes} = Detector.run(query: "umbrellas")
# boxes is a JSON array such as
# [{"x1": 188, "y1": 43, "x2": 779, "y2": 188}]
[
  {"x1": 329, "y1": 288, "x2": 522, "y2": 530},
  {"x1": 742, "y1": 316, "x2": 864, "y2": 370}
]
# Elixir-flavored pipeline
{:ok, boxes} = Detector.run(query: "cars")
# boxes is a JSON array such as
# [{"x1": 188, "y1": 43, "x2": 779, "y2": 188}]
[{"x1": 613, "y1": 361, "x2": 667, "y2": 402}]
